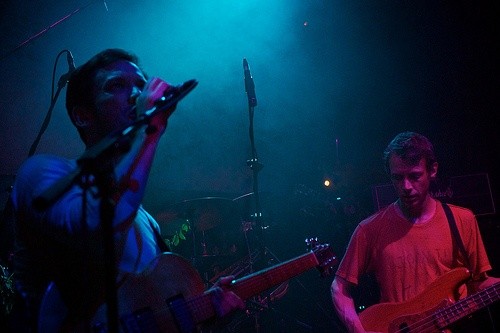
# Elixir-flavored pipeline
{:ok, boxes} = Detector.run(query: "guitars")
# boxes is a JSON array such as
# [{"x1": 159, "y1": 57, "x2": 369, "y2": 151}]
[
  {"x1": 359, "y1": 268, "x2": 500, "y2": 333},
  {"x1": 40, "y1": 237, "x2": 338, "y2": 333}
]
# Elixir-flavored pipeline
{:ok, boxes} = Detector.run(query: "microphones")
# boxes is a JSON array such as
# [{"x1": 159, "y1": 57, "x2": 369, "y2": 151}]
[
  {"x1": 64, "y1": 50, "x2": 76, "y2": 75},
  {"x1": 158, "y1": 80, "x2": 195, "y2": 107},
  {"x1": 242, "y1": 58, "x2": 258, "y2": 106}
]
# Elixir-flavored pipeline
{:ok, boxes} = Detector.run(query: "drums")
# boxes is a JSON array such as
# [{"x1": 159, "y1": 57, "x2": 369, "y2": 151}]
[{"x1": 175, "y1": 197, "x2": 234, "y2": 275}]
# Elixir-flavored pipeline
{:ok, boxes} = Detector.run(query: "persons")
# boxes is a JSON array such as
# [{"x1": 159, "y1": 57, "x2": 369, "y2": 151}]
[
  {"x1": 329, "y1": 131, "x2": 500, "y2": 333},
  {"x1": 0, "y1": 47, "x2": 247, "y2": 333}
]
